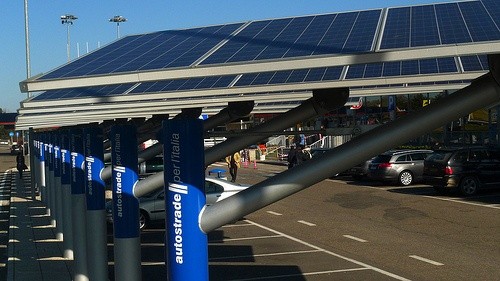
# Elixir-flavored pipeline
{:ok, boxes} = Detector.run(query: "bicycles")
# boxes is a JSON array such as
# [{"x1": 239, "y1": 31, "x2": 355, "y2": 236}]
[{"x1": 16, "y1": 159, "x2": 29, "y2": 178}]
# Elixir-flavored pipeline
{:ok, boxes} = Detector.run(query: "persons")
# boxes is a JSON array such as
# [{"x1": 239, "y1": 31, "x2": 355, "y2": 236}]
[
  {"x1": 225, "y1": 152, "x2": 240, "y2": 183},
  {"x1": 323, "y1": 118, "x2": 329, "y2": 128},
  {"x1": 288, "y1": 146, "x2": 308, "y2": 167},
  {"x1": 16, "y1": 151, "x2": 27, "y2": 178},
  {"x1": 299, "y1": 134, "x2": 306, "y2": 147}
]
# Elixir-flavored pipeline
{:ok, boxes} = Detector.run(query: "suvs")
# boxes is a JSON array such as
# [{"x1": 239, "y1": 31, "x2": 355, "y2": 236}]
[
  {"x1": 310, "y1": 148, "x2": 434, "y2": 186},
  {"x1": 422, "y1": 143, "x2": 500, "y2": 197}
]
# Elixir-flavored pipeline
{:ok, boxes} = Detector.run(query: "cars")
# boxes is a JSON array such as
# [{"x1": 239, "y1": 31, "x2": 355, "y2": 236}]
[
  {"x1": 278, "y1": 147, "x2": 291, "y2": 160},
  {"x1": 10, "y1": 142, "x2": 19, "y2": 154},
  {"x1": 103, "y1": 153, "x2": 248, "y2": 232}
]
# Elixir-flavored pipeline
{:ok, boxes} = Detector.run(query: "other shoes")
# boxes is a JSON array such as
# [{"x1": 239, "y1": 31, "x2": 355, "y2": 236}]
[{"x1": 231, "y1": 179, "x2": 234, "y2": 182}]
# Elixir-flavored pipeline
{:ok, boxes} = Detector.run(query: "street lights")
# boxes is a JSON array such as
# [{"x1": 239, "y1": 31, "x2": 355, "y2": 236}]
[
  {"x1": 109, "y1": 15, "x2": 128, "y2": 39},
  {"x1": 60, "y1": 16, "x2": 78, "y2": 62}
]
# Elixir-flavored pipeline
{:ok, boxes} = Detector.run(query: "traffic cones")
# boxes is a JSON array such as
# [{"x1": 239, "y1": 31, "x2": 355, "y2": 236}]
[
  {"x1": 244, "y1": 159, "x2": 248, "y2": 167},
  {"x1": 253, "y1": 159, "x2": 258, "y2": 169}
]
